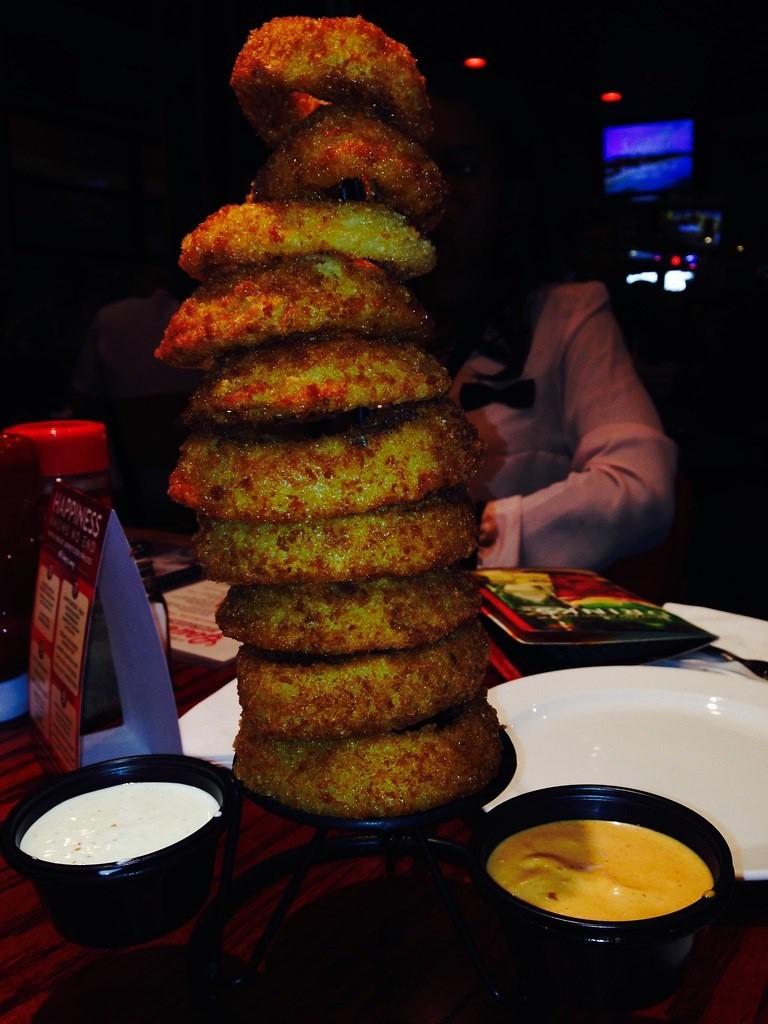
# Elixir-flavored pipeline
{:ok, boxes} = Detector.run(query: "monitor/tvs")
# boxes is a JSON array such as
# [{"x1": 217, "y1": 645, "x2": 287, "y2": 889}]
[{"x1": 604, "y1": 118, "x2": 696, "y2": 205}]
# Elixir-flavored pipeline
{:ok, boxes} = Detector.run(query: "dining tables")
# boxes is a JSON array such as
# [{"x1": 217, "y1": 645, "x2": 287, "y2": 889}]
[{"x1": 0, "y1": 528, "x2": 768, "y2": 1024}]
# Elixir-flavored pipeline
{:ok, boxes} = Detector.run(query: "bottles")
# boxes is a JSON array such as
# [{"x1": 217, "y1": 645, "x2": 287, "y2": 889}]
[{"x1": 1, "y1": 417, "x2": 184, "y2": 772}]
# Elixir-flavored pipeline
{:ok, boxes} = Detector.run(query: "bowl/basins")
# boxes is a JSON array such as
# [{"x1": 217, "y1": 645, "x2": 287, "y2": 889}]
[
  {"x1": 467, "y1": 784, "x2": 736, "y2": 1005},
  {"x1": 1, "y1": 753, "x2": 228, "y2": 946}
]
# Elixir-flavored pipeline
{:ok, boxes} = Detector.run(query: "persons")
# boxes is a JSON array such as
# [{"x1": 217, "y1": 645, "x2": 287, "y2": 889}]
[
  {"x1": 421, "y1": 87, "x2": 680, "y2": 570},
  {"x1": 73, "y1": 258, "x2": 201, "y2": 403}
]
